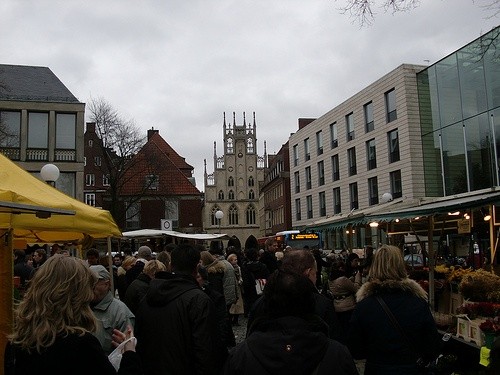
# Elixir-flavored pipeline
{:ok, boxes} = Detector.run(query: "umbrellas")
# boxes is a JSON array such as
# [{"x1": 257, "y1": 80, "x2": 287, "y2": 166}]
[{"x1": 120, "y1": 227, "x2": 165, "y2": 237}]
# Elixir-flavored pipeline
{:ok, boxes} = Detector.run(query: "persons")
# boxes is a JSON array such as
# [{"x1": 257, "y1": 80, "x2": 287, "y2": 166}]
[
  {"x1": 347, "y1": 245, "x2": 443, "y2": 375},
  {"x1": 223, "y1": 270, "x2": 358, "y2": 375},
  {"x1": 13, "y1": 238, "x2": 494, "y2": 355},
  {"x1": 133, "y1": 242, "x2": 232, "y2": 375},
  {"x1": 1, "y1": 254, "x2": 145, "y2": 375}
]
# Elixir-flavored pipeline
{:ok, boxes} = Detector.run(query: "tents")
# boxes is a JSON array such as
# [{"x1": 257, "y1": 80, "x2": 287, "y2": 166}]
[{"x1": 0, "y1": 150, "x2": 124, "y2": 339}]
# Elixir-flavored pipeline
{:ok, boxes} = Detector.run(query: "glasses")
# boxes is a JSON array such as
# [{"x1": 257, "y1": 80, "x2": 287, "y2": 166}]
[{"x1": 28, "y1": 259, "x2": 34, "y2": 261}]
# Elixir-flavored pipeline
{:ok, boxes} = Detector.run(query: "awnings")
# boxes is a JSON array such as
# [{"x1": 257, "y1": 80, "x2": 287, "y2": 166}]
[
  {"x1": 363, "y1": 191, "x2": 500, "y2": 226},
  {"x1": 275, "y1": 230, "x2": 300, "y2": 236},
  {"x1": 161, "y1": 230, "x2": 234, "y2": 240},
  {"x1": 306, "y1": 199, "x2": 421, "y2": 233}
]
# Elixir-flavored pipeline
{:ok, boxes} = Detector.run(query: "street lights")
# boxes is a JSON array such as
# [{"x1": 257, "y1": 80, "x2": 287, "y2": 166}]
[
  {"x1": 215, "y1": 211, "x2": 224, "y2": 251},
  {"x1": 40, "y1": 163, "x2": 59, "y2": 259}
]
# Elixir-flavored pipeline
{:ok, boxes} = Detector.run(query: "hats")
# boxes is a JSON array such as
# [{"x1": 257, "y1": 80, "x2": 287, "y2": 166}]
[
  {"x1": 170, "y1": 244, "x2": 201, "y2": 273},
  {"x1": 137, "y1": 245, "x2": 153, "y2": 261},
  {"x1": 24, "y1": 255, "x2": 32, "y2": 262},
  {"x1": 89, "y1": 265, "x2": 110, "y2": 279}
]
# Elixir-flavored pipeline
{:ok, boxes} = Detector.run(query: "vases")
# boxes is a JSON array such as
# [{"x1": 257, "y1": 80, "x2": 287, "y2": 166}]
[{"x1": 484, "y1": 333, "x2": 494, "y2": 349}]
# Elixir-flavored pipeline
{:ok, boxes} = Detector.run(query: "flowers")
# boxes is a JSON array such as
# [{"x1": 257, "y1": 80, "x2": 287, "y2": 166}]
[{"x1": 435, "y1": 265, "x2": 500, "y2": 333}]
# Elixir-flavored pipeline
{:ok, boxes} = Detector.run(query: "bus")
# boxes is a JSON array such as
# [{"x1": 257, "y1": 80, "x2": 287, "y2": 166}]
[{"x1": 256, "y1": 233, "x2": 321, "y2": 250}]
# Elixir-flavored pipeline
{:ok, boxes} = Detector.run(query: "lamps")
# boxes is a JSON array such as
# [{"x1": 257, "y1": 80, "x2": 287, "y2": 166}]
[{"x1": 480, "y1": 207, "x2": 491, "y2": 221}]
